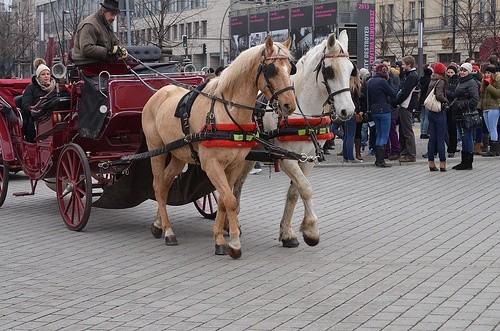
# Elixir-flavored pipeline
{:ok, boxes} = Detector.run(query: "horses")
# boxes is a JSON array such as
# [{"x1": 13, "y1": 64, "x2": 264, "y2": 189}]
[
  {"x1": 138, "y1": 35, "x2": 296, "y2": 259},
  {"x1": 223, "y1": 30, "x2": 356, "y2": 249}
]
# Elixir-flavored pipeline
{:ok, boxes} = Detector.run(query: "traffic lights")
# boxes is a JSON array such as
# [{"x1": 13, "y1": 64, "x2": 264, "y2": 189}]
[
  {"x1": 203, "y1": 43, "x2": 207, "y2": 55},
  {"x1": 182, "y1": 35, "x2": 187, "y2": 48}
]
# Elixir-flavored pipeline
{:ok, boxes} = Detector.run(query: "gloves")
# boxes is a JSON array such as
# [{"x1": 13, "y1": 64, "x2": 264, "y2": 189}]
[
  {"x1": 108, "y1": 46, "x2": 122, "y2": 56},
  {"x1": 120, "y1": 47, "x2": 128, "y2": 58},
  {"x1": 483, "y1": 80, "x2": 489, "y2": 87}
]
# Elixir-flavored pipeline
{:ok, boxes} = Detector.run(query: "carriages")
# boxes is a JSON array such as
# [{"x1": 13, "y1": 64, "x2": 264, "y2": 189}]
[{"x1": 0, "y1": 29, "x2": 363, "y2": 259}]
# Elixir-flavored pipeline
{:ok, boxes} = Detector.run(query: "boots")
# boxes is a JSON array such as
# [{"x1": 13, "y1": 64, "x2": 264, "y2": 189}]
[
  {"x1": 374, "y1": 145, "x2": 379, "y2": 165},
  {"x1": 355, "y1": 146, "x2": 364, "y2": 160},
  {"x1": 378, "y1": 145, "x2": 392, "y2": 168}
]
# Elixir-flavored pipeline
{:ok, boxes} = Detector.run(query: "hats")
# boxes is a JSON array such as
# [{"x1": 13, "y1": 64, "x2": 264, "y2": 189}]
[
  {"x1": 100, "y1": 0, "x2": 121, "y2": 13},
  {"x1": 34, "y1": 58, "x2": 50, "y2": 77},
  {"x1": 472, "y1": 65, "x2": 479, "y2": 72},
  {"x1": 446, "y1": 62, "x2": 457, "y2": 74},
  {"x1": 434, "y1": 63, "x2": 446, "y2": 73},
  {"x1": 461, "y1": 63, "x2": 472, "y2": 73},
  {"x1": 359, "y1": 68, "x2": 369, "y2": 79},
  {"x1": 485, "y1": 67, "x2": 494, "y2": 73}
]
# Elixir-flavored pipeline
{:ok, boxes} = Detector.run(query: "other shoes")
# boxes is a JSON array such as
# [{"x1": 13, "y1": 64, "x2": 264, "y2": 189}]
[
  {"x1": 337, "y1": 150, "x2": 343, "y2": 155},
  {"x1": 420, "y1": 134, "x2": 430, "y2": 139},
  {"x1": 422, "y1": 141, "x2": 499, "y2": 172},
  {"x1": 327, "y1": 147, "x2": 335, "y2": 149},
  {"x1": 348, "y1": 159, "x2": 358, "y2": 163},
  {"x1": 250, "y1": 168, "x2": 262, "y2": 174},
  {"x1": 399, "y1": 156, "x2": 416, "y2": 162},
  {"x1": 324, "y1": 149, "x2": 330, "y2": 154},
  {"x1": 389, "y1": 154, "x2": 398, "y2": 160}
]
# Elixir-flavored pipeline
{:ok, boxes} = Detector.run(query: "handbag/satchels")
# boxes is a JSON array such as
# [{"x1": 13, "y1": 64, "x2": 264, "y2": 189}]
[
  {"x1": 424, "y1": 79, "x2": 441, "y2": 112},
  {"x1": 462, "y1": 101, "x2": 482, "y2": 128},
  {"x1": 397, "y1": 89, "x2": 412, "y2": 108}
]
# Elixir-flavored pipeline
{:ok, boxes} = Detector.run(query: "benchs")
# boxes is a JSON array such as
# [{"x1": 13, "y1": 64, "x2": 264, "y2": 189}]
[
  {"x1": 14, "y1": 91, "x2": 71, "y2": 143},
  {"x1": 67, "y1": 45, "x2": 180, "y2": 96}
]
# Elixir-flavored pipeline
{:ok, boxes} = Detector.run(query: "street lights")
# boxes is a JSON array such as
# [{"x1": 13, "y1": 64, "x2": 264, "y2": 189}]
[{"x1": 61, "y1": 9, "x2": 71, "y2": 57}]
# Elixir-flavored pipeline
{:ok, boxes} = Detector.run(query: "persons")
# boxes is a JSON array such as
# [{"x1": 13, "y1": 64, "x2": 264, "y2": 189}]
[
  {"x1": 480, "y1": 64, "x2": 500, "y2": 157},
  {"x1": 365, "y1": 64, "x2": 396, "y2": 167},
  {"x1": 426, "y1": 63, "x2": 449, "y2": 172},
  {"x1": 252, "y1": 33, "x2": 266, "y2": 46},
  {"x1": 21, "y1": 58, "x2": 56, "y2": 143},
  {"x1": 240, "y1": 38, "x2": 245, "y2": 49},
  {"x1": 322, "y1": 58, "x2": 500, "y2": 163},
  {"x1": 72, "y1": 0, "x2": 128, "y2": 66},
  {"x1": 207, "y1": 67, "x2": 261, "y2": 174},
  {"x1": 390, "y1": 55, "x2": 421, "y2": 162},
  {"x1": 303, "y1": 42, "x2": 309, "y2": 55},
  {"x1": 451, "y1": 62, "x2": 479, "y2": 169}
]
